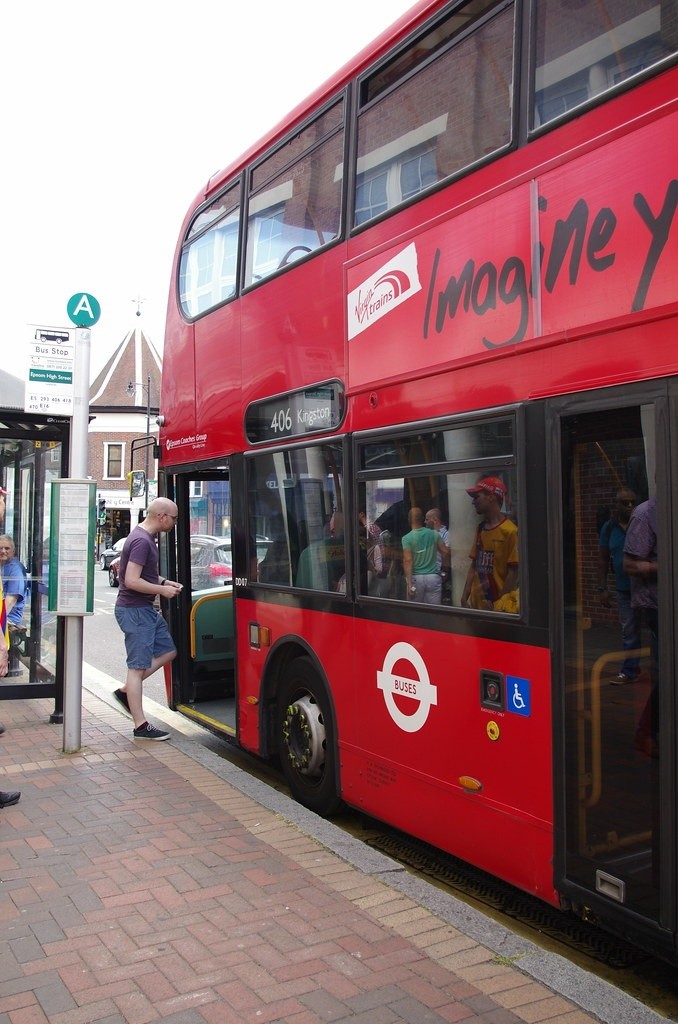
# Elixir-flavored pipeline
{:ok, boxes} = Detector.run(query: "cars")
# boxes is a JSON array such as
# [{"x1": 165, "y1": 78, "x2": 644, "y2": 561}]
[
  {"x1": 100, "y1": 537, "x2": 158, "y2": 570},
  {"x1": 108, "y1": 543, "x2": 159, "y2": 587}
]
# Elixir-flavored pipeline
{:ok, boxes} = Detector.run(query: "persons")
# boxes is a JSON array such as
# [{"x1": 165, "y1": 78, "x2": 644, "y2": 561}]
[
  {"x1": 596, "y1": 487, "x2": 654, "y2": 685},
  {"x1": 622, "y1": 499, "x2": 660, "y2": 758},
  {"x1": 461, "y1": 477, "x2": 519, "y2": 614},
  {"x1": 114, "y1": 497, "x2": 183, "y2": 740},
  {"x1": 359, "y1": 503, "x2": 385, "y2": 597},
  {"x1": 401, "y1": 507, "x2": 449, "y2": 605},
  {"x1": 0, "y1": 486, "x2": 25, "y2": 808},
  {"x1": 328, "y1": 511, "x2": 336, "y2": 536},
  {"x1": 424, "y1": 509, "x2": 449, "y2": 576}
]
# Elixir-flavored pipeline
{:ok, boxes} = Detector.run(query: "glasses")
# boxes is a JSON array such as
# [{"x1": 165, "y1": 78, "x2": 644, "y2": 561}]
[
  {"x1": 166, "y1": 512, "x2": 178, "y2": 522},
  {"x1": 617, "y1": 499, "x2": 638, "y2": 507}
]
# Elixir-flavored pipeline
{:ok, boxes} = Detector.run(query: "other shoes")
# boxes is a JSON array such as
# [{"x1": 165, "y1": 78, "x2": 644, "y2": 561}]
[
  {"x1": 0, "y1": 789, "x2": 21, "y2": 808},
  {"x1": 634, "y1": 731, "x2": 660, "y2": 759}
]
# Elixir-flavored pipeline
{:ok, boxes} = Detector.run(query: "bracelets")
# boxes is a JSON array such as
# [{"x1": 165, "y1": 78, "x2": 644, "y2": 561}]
[{"x1": 161, "y1": 578, "x2": 168, "y2": 585}]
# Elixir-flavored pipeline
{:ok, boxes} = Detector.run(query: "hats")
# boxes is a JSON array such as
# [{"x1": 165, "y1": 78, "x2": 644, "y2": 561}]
[{"x1": 466, "y1": 477, "x2": 512, "y2": 506}]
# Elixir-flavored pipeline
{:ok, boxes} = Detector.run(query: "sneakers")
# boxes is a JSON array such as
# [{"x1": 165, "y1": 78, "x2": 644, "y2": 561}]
[
  {"x1": 113, "y1": 689, "x2": 131, "y2": 717},
  {"x1": 134, "y1": 724, "x2": 171, "y2": 741},
  {"x1": 609, "y1": 672, "x2": 640, "y2": 685}
]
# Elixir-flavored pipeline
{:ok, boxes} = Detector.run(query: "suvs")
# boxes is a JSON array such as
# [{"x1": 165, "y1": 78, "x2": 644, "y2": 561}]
[{"x1": 175, "y1": 532, "x2": 274, "y2": 591}]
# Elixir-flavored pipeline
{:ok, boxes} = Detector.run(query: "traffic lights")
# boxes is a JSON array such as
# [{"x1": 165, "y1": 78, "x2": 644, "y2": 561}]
[{"x1": 97, "y1": 498, "x2": 106, "y2": 525}]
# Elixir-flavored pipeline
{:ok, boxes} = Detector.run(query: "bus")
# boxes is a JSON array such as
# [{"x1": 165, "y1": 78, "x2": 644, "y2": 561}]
[{"x1": 127, "y1": 0, "x2": 678, "y2": 968}]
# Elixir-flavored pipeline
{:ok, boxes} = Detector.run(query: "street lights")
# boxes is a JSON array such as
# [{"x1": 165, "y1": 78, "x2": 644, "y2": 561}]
[{"x1": 125, "y1": 381, "x2": 151, "y2": 509}]
